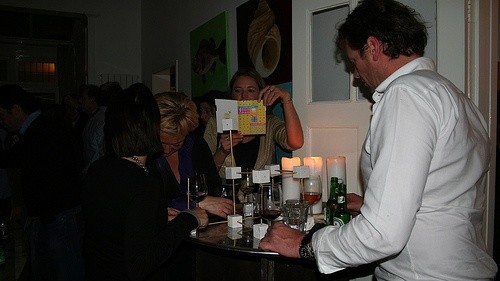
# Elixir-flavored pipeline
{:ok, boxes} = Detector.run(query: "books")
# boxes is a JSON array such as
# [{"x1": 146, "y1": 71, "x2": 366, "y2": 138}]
[{"x1": 215, "y1": 99, "x2": 267, "y2": 136}]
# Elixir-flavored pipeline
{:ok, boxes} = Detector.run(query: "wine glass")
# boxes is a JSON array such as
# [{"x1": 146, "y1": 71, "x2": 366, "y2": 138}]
[
  {"x1": 303, "y1": 175, "x2": 323, "y2": 217},
  {"x1": 185, "y1": 173, "x2": 209, "y2": 208}
]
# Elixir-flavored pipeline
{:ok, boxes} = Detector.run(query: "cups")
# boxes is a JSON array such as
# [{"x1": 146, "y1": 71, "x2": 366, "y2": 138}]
[
  {"x1": 239, "y1": 167, "x2": 256, "y2": 195},
  {"x1": 260, "y1": 184, "x2": 310, "y2": 235}
]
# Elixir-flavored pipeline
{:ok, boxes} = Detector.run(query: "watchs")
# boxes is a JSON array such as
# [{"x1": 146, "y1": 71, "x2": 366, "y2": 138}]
[{"x1": 299, "y1": 233, "x2": 314, "y2": 260}]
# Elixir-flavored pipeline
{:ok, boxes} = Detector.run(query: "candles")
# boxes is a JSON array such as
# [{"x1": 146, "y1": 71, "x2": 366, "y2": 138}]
[
  {"x1": 304, "y1": 156, "x2": 323, "y2": 213},
  {"x1": 281, "y1": 156, "x2": 301, "y2": 202},
  {"x1": 326, "y1": 155, "x2": 346, "y2": 200}
]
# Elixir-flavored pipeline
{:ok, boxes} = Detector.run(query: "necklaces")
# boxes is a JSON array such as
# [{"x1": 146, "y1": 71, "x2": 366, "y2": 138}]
[{"x1": 131, "y1": 155, "x2": 149, "y2": 172}]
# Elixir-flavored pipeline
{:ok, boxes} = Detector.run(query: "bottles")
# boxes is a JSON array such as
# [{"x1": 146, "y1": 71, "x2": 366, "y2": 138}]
[
  {"x1": 333, "y1": 184, "x2": 349, "y2": 227},
  {"x1": 325, "y1": 178, "x2": 338, "y2": 226}
]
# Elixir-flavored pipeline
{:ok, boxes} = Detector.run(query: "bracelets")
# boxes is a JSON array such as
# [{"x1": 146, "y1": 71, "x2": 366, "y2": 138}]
[{"x1": 281, "y1": 98, "x2": 294, "y2": 104}]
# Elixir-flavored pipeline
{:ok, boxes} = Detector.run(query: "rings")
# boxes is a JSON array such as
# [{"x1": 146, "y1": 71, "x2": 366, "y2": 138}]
[
  {"x1": 226, "y1": 137, "x2": 229, "y2": 140},
  {"x1": 268, "y1": 89, "x2": 271, "y2": 92}
]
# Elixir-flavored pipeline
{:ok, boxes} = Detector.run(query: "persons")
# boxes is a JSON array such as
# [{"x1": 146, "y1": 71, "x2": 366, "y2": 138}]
[
  {"x1": 204, "y1": 67, "x2": 304, "y2": 203},
  {"x1": 155, "y1": 92, "x2": 234, "y2": 224},
  {"x1": 190, "y1": 88, "x2": 224, "y2": 133},
  {"x1": 259, "y1": 0, "x2": 498, "y2": 281},
  {"x1": 87, "y1": 83, "x2": 208, "y2": 281},
  {"x1": 0, "y1": 81, "x2": 122, "y2": 281}
]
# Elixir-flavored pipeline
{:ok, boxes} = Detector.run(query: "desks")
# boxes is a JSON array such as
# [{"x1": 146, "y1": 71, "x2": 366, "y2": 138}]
[{"x1": 184, "y1": 204, "x2": 357, "y2": 281}]
